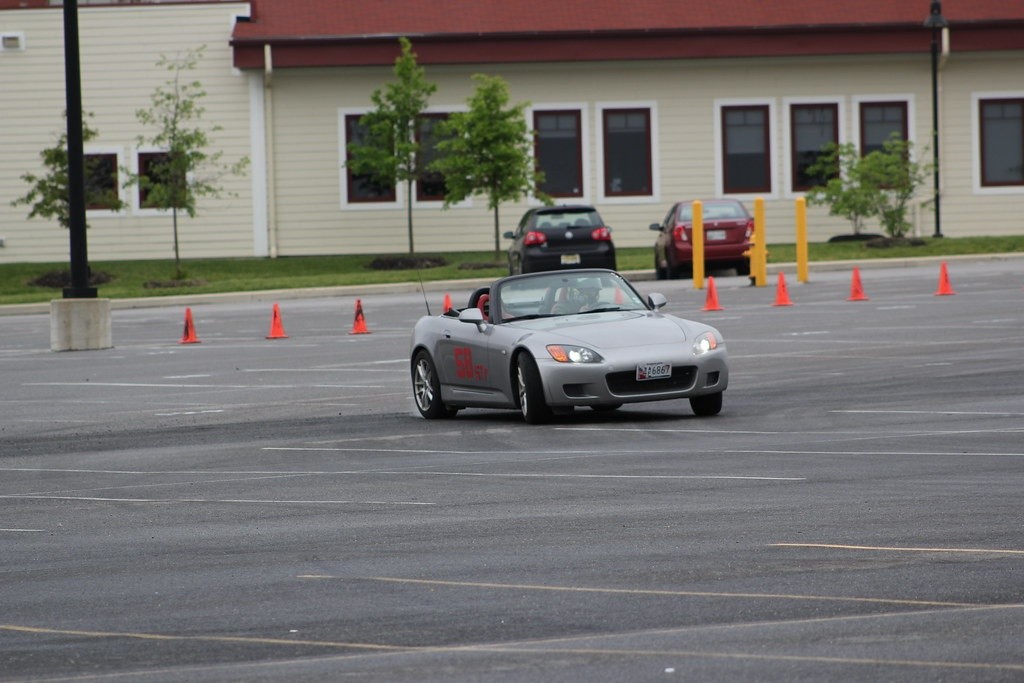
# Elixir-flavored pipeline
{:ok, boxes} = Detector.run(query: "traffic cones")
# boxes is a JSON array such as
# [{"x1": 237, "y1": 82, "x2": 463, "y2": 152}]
[
  {"x1": 610, "y1": 283, "x2": 624, "y2": 306},
  {"x1": 699, "y1": 276, "x2": 725, "y2": 313},
  {"x1": 770, "y1": 271, "x2": 796, "y2": 308},
  {"x1": 442, "y1": 292, "x2": 453, "y2": 314},
  {"x1": 348, "y1": 299, "x2": 372, "y2": 334},
  {"x1": 265, "y1": 304, "x2": 288, "y2": 338},
  {"x1": 177, "y1": 307, "x2": 205, "y2": 344},
  {"x1": 935, "y1": 260, "x2": 956, "y2": 297},
  {"x1": 845, "y1": 266, "x2": 871, "y2": 302}
]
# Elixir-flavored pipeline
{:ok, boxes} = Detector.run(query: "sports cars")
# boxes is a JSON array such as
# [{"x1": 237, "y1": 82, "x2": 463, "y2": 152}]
[{"x1": 410, "y1": 266, "x2": 731, "y2": 422}]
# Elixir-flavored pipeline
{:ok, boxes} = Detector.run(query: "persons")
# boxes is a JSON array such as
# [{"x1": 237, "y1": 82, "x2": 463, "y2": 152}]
[{"x1": 553, "y1": 278, "x2": 603, "y2": 314}]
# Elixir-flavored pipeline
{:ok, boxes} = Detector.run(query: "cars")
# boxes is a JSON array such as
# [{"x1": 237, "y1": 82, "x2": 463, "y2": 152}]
[
  {"x1": 503, "y1": 205, "x2": 618, "y2": 281},
  {"x1": 649, "y1": 198, "x2": 754, "y2": 278}
]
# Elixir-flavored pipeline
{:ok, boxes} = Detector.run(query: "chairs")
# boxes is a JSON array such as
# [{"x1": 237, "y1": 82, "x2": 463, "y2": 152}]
[
  {"x1": 468, "y1": 286, "x2": 520, "y2": 322},
  {"x1": 541, "y1": 282, "x2": 582, "y2": 314}
]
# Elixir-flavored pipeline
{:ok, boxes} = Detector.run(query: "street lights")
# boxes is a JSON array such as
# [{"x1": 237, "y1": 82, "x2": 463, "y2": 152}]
[{"x1": 923, "y1": 1, "x2": 948, "y2": 238}]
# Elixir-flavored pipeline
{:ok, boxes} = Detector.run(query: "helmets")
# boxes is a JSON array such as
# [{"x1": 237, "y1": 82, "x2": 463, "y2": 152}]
[{"x1": 566, "y1": 277, "x2": 603, "y2": 305}]
